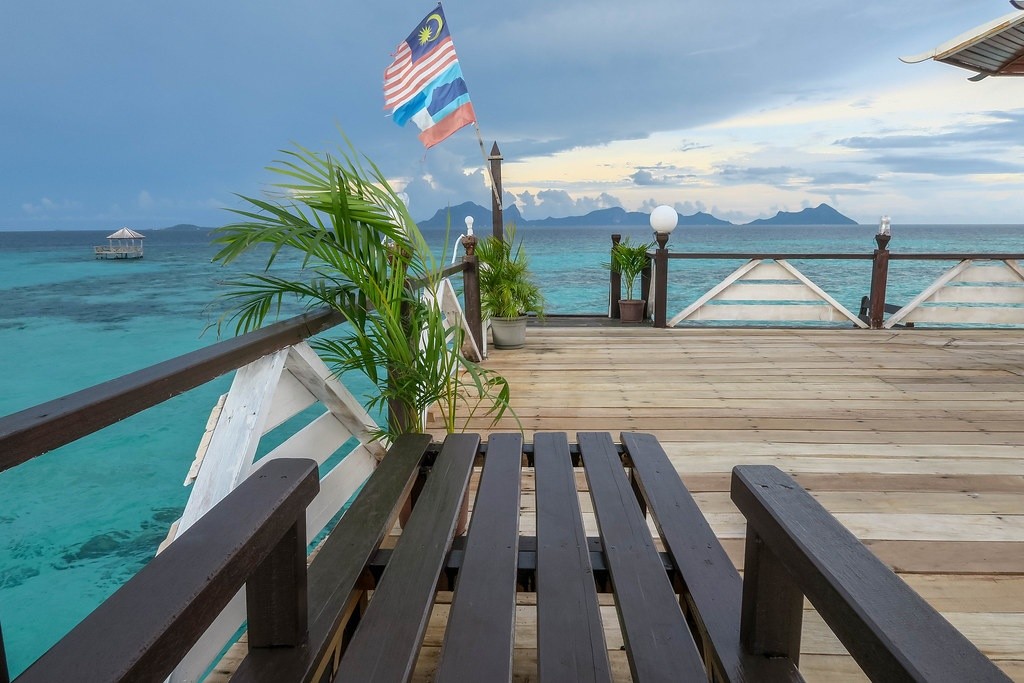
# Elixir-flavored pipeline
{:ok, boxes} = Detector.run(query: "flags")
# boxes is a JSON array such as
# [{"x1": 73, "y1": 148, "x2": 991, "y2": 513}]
[{"x1": 380, "y1": 3, "x2": 474, "y2": 148}]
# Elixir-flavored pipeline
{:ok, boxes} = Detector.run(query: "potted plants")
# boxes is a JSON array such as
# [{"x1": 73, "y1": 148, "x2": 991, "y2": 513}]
[
  {"x1": 599, "y1": 233, "x2": 658, "y2": 323},
  {"x1": 471, "y1": 219, "x2": 546, "y2": 350},
  {"x1": 208, "y1": 114, "x2": 525, "y2": 540}
]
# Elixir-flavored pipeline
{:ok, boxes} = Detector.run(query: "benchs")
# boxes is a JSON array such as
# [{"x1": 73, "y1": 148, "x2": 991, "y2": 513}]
[{"x1": 3, "y1": 432, "x2": 1018, "y2": 683}]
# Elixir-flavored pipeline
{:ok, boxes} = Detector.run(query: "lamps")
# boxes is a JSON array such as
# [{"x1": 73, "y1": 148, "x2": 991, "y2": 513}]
[{"x1": 649, "y1": 205, "x2": 679, "y2": 249}]
